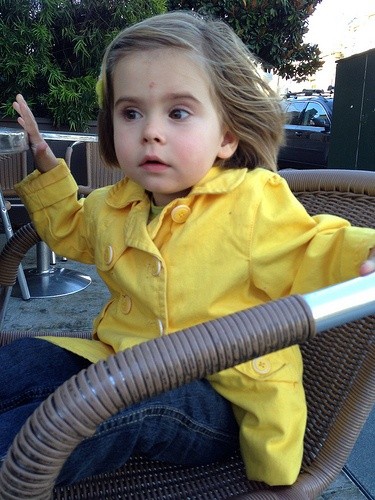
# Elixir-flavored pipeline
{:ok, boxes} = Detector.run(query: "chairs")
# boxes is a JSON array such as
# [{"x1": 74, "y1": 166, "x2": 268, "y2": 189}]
[
  {"x1": 0, "y1": 149, "x2": 29, "y2": 206},
  {"x1": 2, "y1": 166, "x2": 374, "y2": 498},
  {"x1": 60, "y1": 140, "x2": 135, "y2": 201}
]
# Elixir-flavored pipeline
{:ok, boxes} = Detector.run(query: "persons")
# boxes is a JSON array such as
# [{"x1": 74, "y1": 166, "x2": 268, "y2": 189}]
[{"x1": 1, "y1": 10, "x2": 375, "y2": 486}]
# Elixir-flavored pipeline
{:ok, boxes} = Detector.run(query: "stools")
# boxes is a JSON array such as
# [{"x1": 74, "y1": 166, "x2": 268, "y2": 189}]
[{"x1": 2, "y1": 138, "x2": 93, "y2": 298}]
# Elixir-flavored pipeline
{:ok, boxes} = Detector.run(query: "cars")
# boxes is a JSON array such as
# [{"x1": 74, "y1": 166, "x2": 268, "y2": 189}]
[{"x1": 276, "y1": 89, "x2": 333, "y2": 167}]
